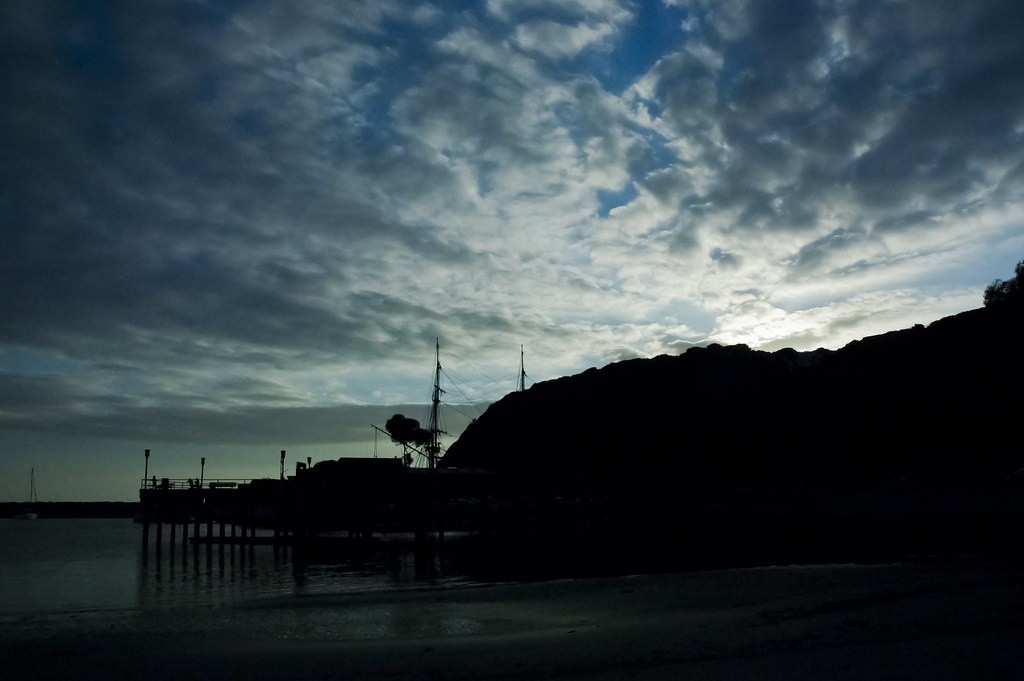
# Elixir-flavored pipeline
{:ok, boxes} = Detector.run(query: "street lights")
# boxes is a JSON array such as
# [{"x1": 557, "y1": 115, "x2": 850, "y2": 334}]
[
  {"x1": 281, "y1": 451, "x2": 285, "y2": 480},
  {"x1": 201, "y1": 458, "x2": 205, "y2": 484},
  {"x1": 308, "y1": 457, "x2": 311, "y2": 469},
  {"x1": 145, "y1": 450, "x2": 150, "y2": 489}
]
